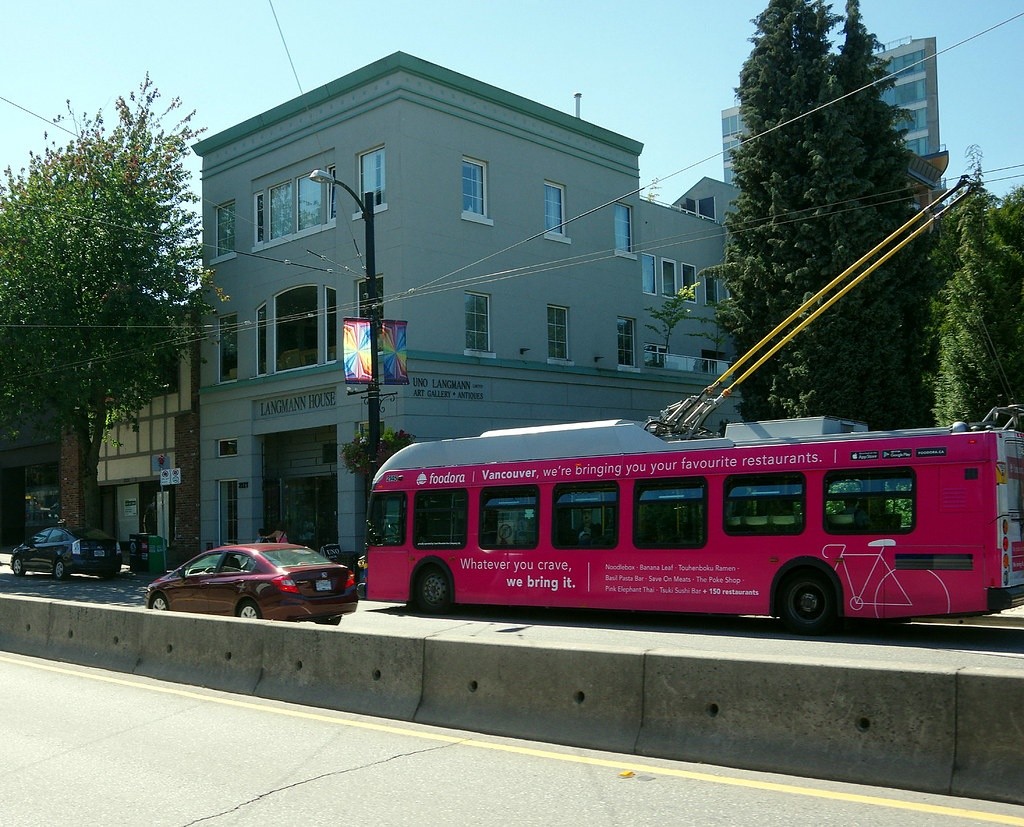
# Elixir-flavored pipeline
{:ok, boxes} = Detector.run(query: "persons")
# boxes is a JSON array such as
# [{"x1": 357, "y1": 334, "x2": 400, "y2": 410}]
[{"x1": 255, "y1": 523, "x2": 288, "y2": 543}]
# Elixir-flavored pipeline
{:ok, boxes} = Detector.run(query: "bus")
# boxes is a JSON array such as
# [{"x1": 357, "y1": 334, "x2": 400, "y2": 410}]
[{"x1": 364, "y1": 405, "x2": 1024, "y2": 632}]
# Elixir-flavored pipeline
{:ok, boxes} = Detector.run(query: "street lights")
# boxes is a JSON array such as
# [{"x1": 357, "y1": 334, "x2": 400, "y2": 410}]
[{"x1": 308, "y1": 168, "x2": 383, "y2": 487}]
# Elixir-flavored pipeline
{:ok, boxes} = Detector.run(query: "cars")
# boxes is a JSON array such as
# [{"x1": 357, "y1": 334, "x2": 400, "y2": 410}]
[
  {"x1": 146, "y1": 542, "x2": 358, "y2": 625},
  {"x1": 9, "y1": 525, "x2": 123, "y2": 580}
]
